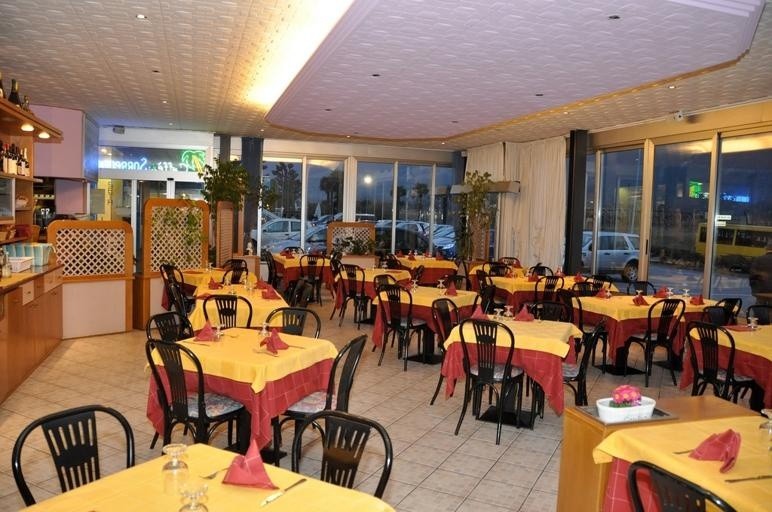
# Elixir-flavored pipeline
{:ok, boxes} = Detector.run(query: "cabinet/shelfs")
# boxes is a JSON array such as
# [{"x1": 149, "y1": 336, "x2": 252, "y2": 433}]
[{"x1": 0, "y1": 98, "x2": 63, "y2": 406}]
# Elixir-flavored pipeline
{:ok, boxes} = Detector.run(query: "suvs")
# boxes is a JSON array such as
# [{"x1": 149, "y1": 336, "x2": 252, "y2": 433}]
[{"x1": 578, "y1": 228, "x2": 653, "y2": 284}]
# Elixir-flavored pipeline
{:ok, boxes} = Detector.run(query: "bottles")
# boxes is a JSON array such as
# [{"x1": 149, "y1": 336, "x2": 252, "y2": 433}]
[
  {"x1": 0, "y1": 70, "x2": 35, "y2": 115},
  {"x1": 0, "y1": 140, "x2": 29, "y2": 177},
  {"x1": 1, "y1": 246, "x2": 12, "y2": 280}
]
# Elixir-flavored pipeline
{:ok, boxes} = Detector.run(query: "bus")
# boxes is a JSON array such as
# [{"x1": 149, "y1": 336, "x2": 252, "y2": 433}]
[{"x1": 693, "y1": 217, "x2": 772, "y2": 271}]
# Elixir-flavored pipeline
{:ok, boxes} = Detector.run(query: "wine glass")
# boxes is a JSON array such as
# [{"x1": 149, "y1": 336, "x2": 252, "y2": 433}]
[
  {"x1": 371, "y1": 262, "x2": 388, "y2": 271},
  {"x1": 410, "y1": 278, "x2": 446, "y2": 293},
  {"x1": 759, "y1": 407, "x2": 772, "y2": 459},
  {"x1": 492, "y1": 306, "x2": 514, "y2": 322},
  {"x1": 290, "y1": 248, "x2": 428, "y2": 261},
  {"x1": 604, "y1": 288, "x2": 692, "y2": 304},
  {"x1": 745, "y1": 316, "x2": 759, "y2": 337},
  {"x1": 205, "y1": 262, "x2": 271, "y2": 344},
  {"x1": 161, "y1": 442, "x2": 211, "y2": 512}
]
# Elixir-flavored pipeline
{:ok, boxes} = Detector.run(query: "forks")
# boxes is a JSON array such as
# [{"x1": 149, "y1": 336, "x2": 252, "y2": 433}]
[{"x1": 201, "y1": 464, "x2": 228, "y2": 480}]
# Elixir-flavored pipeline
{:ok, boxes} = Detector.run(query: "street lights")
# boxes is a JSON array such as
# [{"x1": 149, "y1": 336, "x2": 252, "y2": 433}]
[{"x1": 364, "y1": 174, "x2": 376, "y2": 215}]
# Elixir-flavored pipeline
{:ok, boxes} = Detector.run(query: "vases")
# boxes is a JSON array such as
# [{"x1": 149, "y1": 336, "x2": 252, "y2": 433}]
[{"x1": 596, "y1": 395, "x2": 656, "y2": 423}]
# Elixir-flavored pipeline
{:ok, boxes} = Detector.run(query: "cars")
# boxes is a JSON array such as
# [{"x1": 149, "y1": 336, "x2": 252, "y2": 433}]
[{"x1": 247, "y1": 202, "x2": 497, "y2": 265}]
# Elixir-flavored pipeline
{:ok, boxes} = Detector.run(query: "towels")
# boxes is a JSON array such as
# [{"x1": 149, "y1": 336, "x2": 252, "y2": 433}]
[
  {"x1": 196, "y1": 291, "x2": 213, "y2": 301},
  {"x1": 689, "y1": 429, "x2": 741, "y2": 472},
  {"x1": 221, "y1": 439, "x2": 278, "y2": 489},
  {"x1": 195, "y1": 321, "x2": 219, "y2": 341},
  {"x1": 254, "y1": 278, "x2": 271, "y2": 289},
  {"x1": 207, "y1": 277, "x2": 223, "y2": 290}
]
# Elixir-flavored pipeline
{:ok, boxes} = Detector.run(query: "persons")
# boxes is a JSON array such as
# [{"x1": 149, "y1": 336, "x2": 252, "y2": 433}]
[{"x1": 749, "y1": 238, "x2": 772, "y2": 325}]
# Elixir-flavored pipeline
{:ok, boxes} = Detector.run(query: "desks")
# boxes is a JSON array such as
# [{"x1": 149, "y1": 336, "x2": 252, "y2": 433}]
[{"x1": 556, "y1": 395, "x2": 761, "y2": 512}]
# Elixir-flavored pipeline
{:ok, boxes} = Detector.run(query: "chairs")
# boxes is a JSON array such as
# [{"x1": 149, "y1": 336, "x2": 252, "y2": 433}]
[
  {"x1": 430, "y1": 298, "x2": 460, "y2": 405},
  {"x1": 454, "y1": 318, "x2": 525, "y2": 444},
  {"x1": 159, "y1": 264, "x2": 195, "y2": 313},
  {"x1": 528, "y1": 317, "x2": 608, "y2": 431},
  {"x1": 12, "y1": 404, "x2": 134, "y2": 506},
  {"x1": 628, "y1": 460, "x2": 735, "y2": 512},
  {"x1": 168, "y1": 282, "x2": 196, "y2": 339},
  {"x1": 221, "y1": 259, "x2": 247, "y2": 268},
  {"x1": 202, "y1": 294, "x2": 252, "y2": 328},
  {"x1": 686, "y1": 321, "x2": 764, "y2": 412},
  {"x1": 273, "y1": 333, "x2": 368, "y2": 471},
  {"x1": 145, "y1": 337, "x2": 250, "y2": 455},
  {"x1": 222, "y1": 267, "x2": 249, "y2": 285},
  {"x1": 291, "y1": 409, "x2": 393, "y2": 499},
  {"x1": 146, "y1": 311, "x2": 194, "y2": 341}
]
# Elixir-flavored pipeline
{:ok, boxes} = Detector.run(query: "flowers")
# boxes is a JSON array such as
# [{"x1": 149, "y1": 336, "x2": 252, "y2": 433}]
[{"x1": 609, "y1": 384, "x2": 642, "y2": 405}]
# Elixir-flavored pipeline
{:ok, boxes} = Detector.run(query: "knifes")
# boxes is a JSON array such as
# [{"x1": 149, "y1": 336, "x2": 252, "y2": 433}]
[{"x1": 259, "y1": 478, "x2": 307, "y2": 508}]
